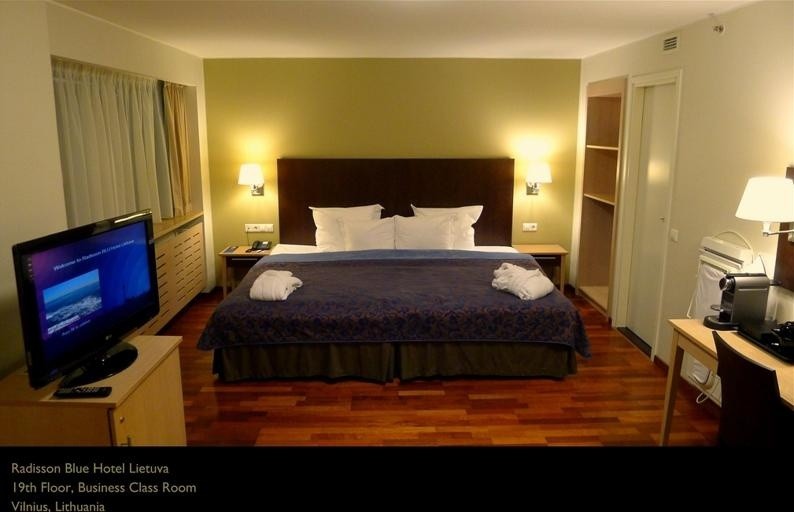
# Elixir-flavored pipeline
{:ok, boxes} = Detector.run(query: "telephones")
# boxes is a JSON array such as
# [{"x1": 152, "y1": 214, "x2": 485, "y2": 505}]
[{"x1": 252, "y1": 240, "x2": 272, "y2": 250}]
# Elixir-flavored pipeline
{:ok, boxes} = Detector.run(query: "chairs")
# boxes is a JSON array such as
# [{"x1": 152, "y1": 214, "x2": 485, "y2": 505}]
[{"x1": 714, "y1": 333, "x2": 786, "y2": 446}]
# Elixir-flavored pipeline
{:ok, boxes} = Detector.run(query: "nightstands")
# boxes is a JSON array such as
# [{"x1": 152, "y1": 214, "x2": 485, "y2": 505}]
[
  {"x1": 219, "y1": 246, "x2": 271, "y2": 299},
  {"x1": 511, "y1": 244, "x2": 569, "y2": 295}
]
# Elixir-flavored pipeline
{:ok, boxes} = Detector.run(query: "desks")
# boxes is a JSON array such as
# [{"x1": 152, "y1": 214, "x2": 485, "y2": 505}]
[{"x1": 658, "y1": 317, "x2": 793, "y2": 446}]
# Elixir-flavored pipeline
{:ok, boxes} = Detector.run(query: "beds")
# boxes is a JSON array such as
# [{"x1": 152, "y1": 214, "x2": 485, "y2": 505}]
[{"x1": 195, "y1": 159, "x2": 593, "y2": 382}]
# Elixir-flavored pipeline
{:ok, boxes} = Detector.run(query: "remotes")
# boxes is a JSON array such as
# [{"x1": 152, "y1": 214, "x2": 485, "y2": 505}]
[{"x1": 54, "y1": 386, "x2": 112, "y2": 397}]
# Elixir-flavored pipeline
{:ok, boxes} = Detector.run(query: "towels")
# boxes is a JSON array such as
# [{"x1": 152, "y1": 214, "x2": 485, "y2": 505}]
[{"x1": 252, "y1": 269, "x2": 303, "y2": 301}]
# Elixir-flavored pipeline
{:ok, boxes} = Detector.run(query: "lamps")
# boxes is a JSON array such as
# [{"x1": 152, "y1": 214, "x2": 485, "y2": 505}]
[
  {"x1": 526, "y1": 164, "x2": 551, "y2": 195},
  {"x1": 734, "y1": 175, "x2": 794, "y2": 242},
  {"x1": 237, "y1": 164, "x2": 263, "y2": 195}
]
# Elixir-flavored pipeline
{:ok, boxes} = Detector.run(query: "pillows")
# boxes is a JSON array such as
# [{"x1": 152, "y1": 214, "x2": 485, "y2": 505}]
[
  {"x1": 409, "y1": 203, "x2": 483, "y2": 252},
  {"x1": 307, "y1": 204, "x2": 384, "y2": 253},
  {"x1": 338, "y1": 216, "x2": 394, "y2": 252},
  {"x1": 393, "y1": 215, "x2": 452, "y2": 251}
]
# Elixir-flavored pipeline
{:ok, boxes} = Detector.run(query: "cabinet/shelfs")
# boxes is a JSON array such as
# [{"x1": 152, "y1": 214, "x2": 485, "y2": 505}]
[
  {"x1": 0, "y1": 335, "x2": 188, "y2": 447},
  {"x1": 579, "y1": 75, "x2": 625, "y2": 312}
]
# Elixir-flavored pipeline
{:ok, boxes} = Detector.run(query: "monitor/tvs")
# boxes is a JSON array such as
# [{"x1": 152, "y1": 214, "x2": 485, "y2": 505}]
[{"x1": 12, "y1": 209, "x2": 160, "y2": 388}]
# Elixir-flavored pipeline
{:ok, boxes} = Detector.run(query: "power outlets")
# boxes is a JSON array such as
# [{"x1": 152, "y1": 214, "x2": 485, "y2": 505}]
[{"x1": 244, "y1": 223, "x2": 251, "y2": 232}]
